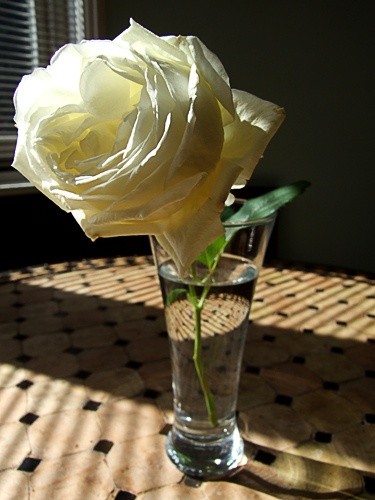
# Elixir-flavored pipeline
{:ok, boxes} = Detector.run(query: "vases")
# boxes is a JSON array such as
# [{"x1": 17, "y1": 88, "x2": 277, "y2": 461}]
[{"x1": 147, "y1": 197, "x2": 279, "y2": 476}]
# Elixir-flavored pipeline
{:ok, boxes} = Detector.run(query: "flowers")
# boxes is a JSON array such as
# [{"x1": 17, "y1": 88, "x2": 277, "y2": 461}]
[{"x1": 10, "y1": 18, "x2": 288, "y2": 277}]
